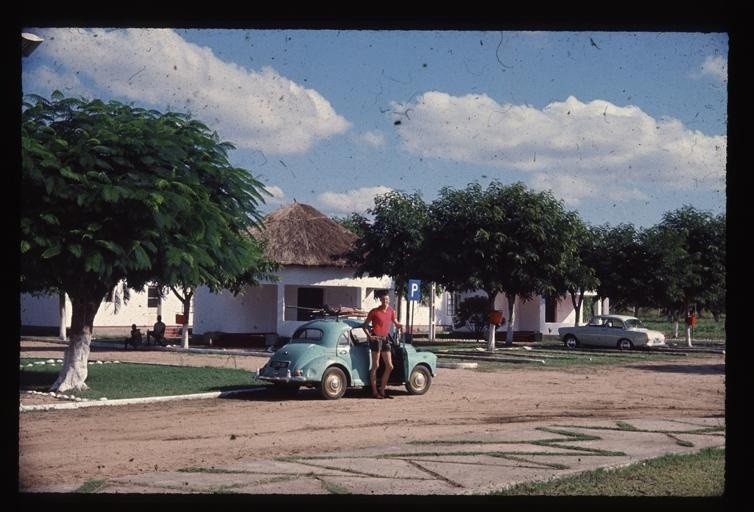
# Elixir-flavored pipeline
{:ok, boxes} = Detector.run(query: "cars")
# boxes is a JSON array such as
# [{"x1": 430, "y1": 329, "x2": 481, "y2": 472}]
[
  {"x1": 252, "y1": 317, "x2": 441, "y2": 403},
  {"x1": 557, "y1": 315, "x2": 666, "y2": 351}
]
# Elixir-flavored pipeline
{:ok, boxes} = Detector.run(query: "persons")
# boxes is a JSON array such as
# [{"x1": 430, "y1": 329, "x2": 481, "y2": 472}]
[
  {"x1": 125, "y1": 325, "x2": 142, "y2": 349},
  {"x1": 363, "y1": 290, "x2": 405, "y2": 398},
  {"x1": 146, "y1": 315, "x2": 165, "y2": 345}
]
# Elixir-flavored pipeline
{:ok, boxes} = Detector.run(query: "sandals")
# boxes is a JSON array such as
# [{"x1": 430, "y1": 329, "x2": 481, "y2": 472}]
[{"x1": 372, "y1": 392, "x2": 389, "y2": 399}]
